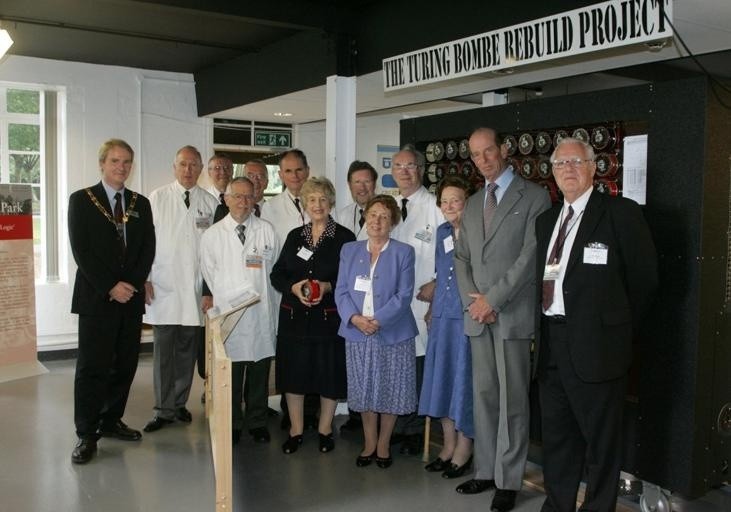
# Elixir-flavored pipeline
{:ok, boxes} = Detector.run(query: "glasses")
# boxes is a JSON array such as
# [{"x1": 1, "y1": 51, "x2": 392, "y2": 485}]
[{"x1": 552, "y1": 158, "x2": 594, "y2": 169}]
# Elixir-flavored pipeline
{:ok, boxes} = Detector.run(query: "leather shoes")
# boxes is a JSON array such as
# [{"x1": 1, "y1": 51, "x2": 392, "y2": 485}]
[
  {"x1": 280, "y1": 416, "x2": 425, "y2": 466},
  {"x1": 143, "y1": 406, "x2": 193, "y2": 432},
  {"x1": 426, "y1": 450, "x2": 518, "y2": 510},
  {"x1": 70, "y1": 439, "x2": 98, "y2": 464},
  {"x1": 197, "y1": 396, "x2": 273, "y2": 444},
  {"x1": 99, "y1": 419, "x2": 141, "y2": 441}
]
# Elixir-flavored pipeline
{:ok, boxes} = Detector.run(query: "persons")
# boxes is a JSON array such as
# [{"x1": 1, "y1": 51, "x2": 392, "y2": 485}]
[
  {"x1": 67, "y1": 138, "x2": 157, "y2": 467},
  {"x1": 145, "y1": 128, "x2": 661, "y2": 512}
]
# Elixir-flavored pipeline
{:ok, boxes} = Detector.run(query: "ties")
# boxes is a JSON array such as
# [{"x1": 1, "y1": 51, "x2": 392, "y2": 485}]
[
  {"x1": 358, "y1": 196, "x2": 408, "y2": 229},
  {"x1": 114, "y1": 191, "x2": 127, "y2": 227},
  {"x1": 543, "y1": 204, "x2": 574, "y2": 311},
  {"x1": 236, "y1": 224, "x2": 245, "y2": 243},
  {"x1": 184, "y1": 190, "x2": 303, "y2": 219},
  {"x1": 485, "y1": 182, "x2": 499, "y2": 238}
]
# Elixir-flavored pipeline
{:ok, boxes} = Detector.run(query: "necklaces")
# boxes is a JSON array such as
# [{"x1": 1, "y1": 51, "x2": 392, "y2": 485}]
[{"x1": 83, "y1": 187, "x2": 138, "y2": 232}]
